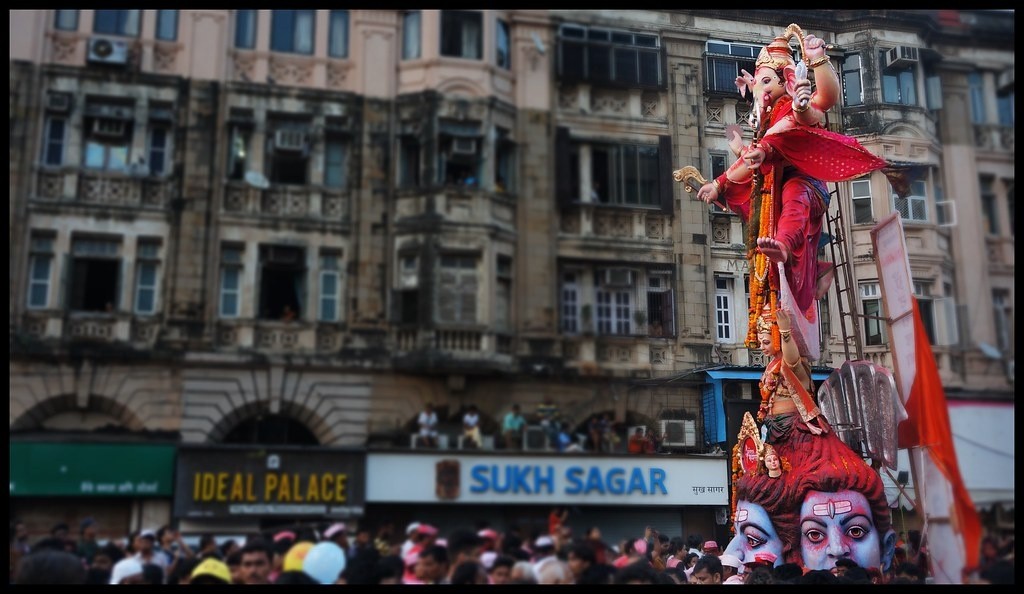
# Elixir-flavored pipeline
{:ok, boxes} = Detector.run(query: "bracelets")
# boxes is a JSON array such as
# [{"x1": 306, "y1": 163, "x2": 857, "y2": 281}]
[
  {"x1": 810, "y1": 54, "x2": 830, "y2": 68},
  {"x1": 791, "y1": 102, "x2": 810, "y2": 113},
  {"x1": 738, "y1": 145, "x2": 745, "y2": 157},
  {"x1": 780, "y1": 329, "x2": 792, "y2": 343},
  {"x1": 713, "y1": 180, "x2": 722, "y2": 194},
  {"x1": 757, "y1": 143, "x2": 771, "y2": 160}
]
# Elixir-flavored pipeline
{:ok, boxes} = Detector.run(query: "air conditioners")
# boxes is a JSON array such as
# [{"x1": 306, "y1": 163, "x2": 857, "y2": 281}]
[
  {"x1": 412, "y1": 434, "x2": 448, "y2": 448},
  {"x1": 886, "y1": 46, "x2": 919, "y2": 70},
  {"x1": 458, "y1": 436, "x2": 494, "y2": 450},
  {"x1": 86, "y1": 35, "x2": 129, "y2": 64},
  {"x1": 655, "y1": 420, "x2": 696, "y2": 446},
  {"x1": 449, "y1": 138, "x2": 476, "y2": 154},
  {"x1": 93, "y1": 119, "x2": 124, "y2": 137},
  {"x1": 522, "y1": 426, "x2": 549, "y2": 450},
  {"x1": 274, "y1": 130, "x2": 303, "y2": 151}
]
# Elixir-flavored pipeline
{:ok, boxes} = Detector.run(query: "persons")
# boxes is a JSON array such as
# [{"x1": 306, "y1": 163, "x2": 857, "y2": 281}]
[
  {"x1": 503, "y1": 405, "x2": 528, "y2": 448},
  {"x1": 462, "y1": 405, "x2": 483, "y2": 449},
  {"x1": 697, "y1": 24, "x2": 899, "y2": 570},
  {"x1": 590, "y1": 413, "x2": 667, "y2": 453},
  {"x1": 11, "y1": 507, "x2": 1016, "y2": 585},
  {"x1": 558, "y1": 423, "x2": 584, "y2": 452},
  {"x1": 418, "y1": 404, "x2": 438, "y2": 447}
]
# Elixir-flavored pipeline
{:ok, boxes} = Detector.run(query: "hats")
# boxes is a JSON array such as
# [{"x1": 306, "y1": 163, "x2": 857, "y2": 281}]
[
  {"x1": 139, "y1": 527, "x2": 155, "y2": 541},
  {"x1": 50, "y1": 521, "x2": 69, "y2": 534},
  {"x1": 188, "y1": 558, "x2": 232, "y2": 584},
  {"x1": 281, "y1": 540, "x2": 346, "y2": 585},
  {"x1": 476, "y1": 529, "x2": 497, "y2": 540},
  {"x1": 703, "y1": 540, "x2": 719, "y2": 552},
  {"x1": 720, "y1": 553, "x2": 741, "y2": 569},
  {"x1": 536, "y1": 537, "x2": 553, "y2": 546},
  {"x1": 102, "y1": 542, "x2": 126, "y2": 563},
  {"x1": 272, "y1": 530, "x2": 296, "y2": 544},
  {"x1": 754, "y1": 551, "x2": 778, "y2": 565},
  {"x1": 78, "y1": 517, "x2": 94, "y2": 533},
  {"x1": 30, "y1": 538, "x2": 65, "y2": 553},
  {"x1": 405, "y1": 521, "x2": 438, "y2": 540}
]
